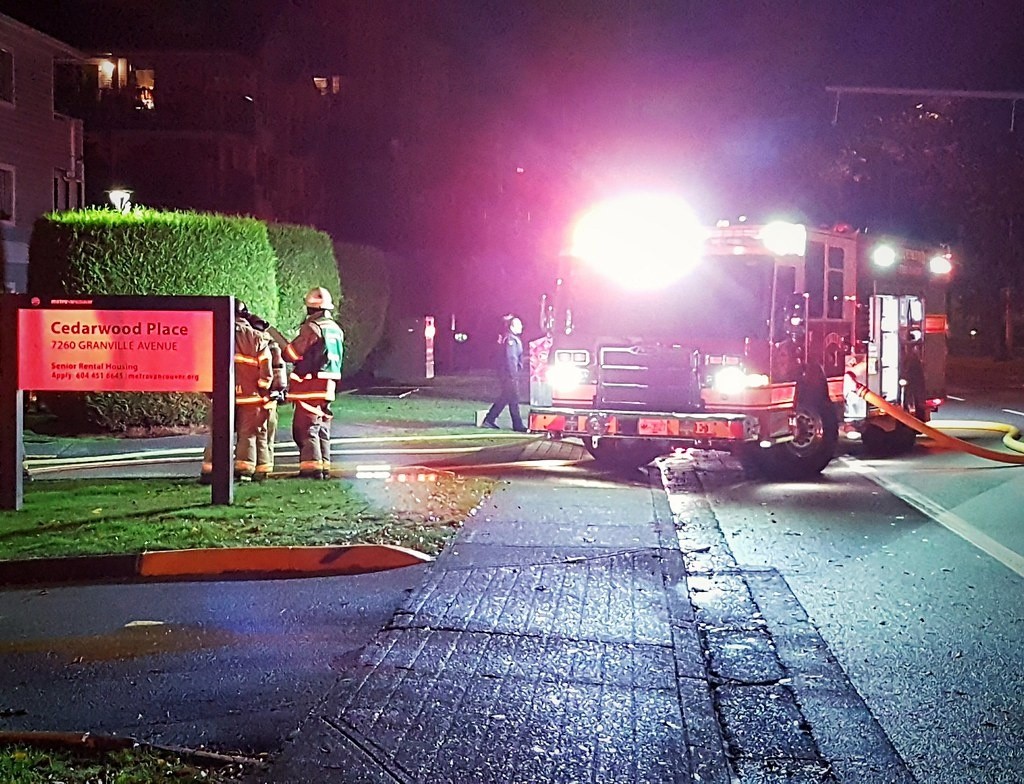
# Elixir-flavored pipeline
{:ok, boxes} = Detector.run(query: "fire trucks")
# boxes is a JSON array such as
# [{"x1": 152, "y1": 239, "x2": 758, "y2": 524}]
[{"x1": 532, "y1": 212, "x2": 951, "y2": 477}]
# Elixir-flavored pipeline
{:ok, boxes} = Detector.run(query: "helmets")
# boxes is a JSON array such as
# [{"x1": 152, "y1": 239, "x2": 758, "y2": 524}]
[
  {"x1": 234, "y1": 299, "x2": 250, "y2": 318},
  {"x1": 305, "y1": 287, "x2": 334, "y2": 309}
]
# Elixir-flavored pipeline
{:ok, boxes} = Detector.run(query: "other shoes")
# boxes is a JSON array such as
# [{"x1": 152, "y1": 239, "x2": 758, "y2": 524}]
[
  {"x1": 288, "y1": 471, "x2": 323, "y2": 479},
  {"x1": 196, "y1": 474, "x2": 211, "y2": 484},
  {"x1": 251, "y1": 472, "x2": 268, "y2": 480},
  {"x1": 233, "y1": 473, "x2": 252, "y2": 482},
  {"x1": 514, "y1": 428, "x2": 527, "y2": 432},
  {"x1": 322, "y1": 471, "x2": 331, "y2": 480},
  {"x1": 481, "y1": 418, "x2": 501, "y2": 429}
]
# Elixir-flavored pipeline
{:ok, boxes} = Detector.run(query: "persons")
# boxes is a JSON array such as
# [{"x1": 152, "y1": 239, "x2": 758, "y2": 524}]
[
  {"x1": 481, "y1": 317, "x2": 530, "y2": 433},
  {"x1": 199, "y1": 298, "x2": 286, "y2": 482},
  {"x1": 282, "y1": 287, "x2": 343, "y2": 480}
]
